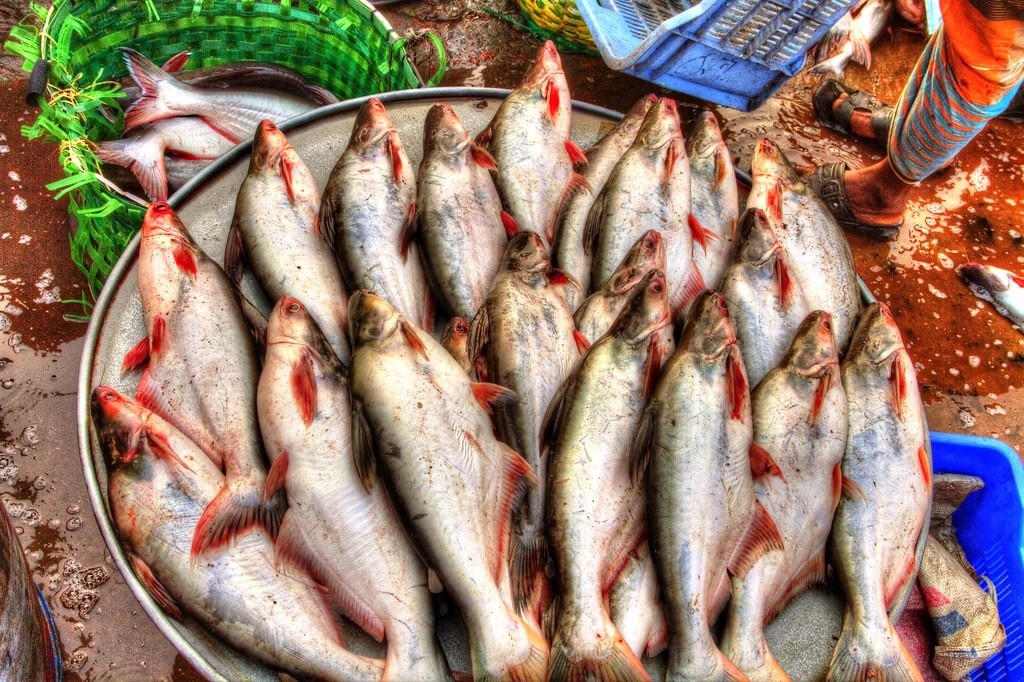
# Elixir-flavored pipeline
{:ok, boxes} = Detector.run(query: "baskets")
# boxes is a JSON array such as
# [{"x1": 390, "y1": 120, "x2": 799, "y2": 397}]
[
  {"x1": 518, "y1": 0, "x2": 601, "y2": 59},
  {"x1": 6, "y1": 0, "x2": 446, "y2": 308},
  {"x1": 576, "y1": 0, "x2": 860, "y2": 113},
  {"x1": 929, "y1": 431, "x2": 1024, "y2": 682}
]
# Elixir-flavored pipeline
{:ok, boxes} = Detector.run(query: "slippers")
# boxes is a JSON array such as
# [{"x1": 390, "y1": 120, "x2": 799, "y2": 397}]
[
  {"x1": 813, "y1": 78, "x2": 957, "y2": 174},
  {"x1": 792, "y1": 162, "x2": 901, "y2": 239}
]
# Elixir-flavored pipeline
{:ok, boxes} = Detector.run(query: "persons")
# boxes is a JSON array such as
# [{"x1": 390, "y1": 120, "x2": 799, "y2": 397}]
[{"x1": 789, "y1": 0, "x2": 1024, "y2": 239}]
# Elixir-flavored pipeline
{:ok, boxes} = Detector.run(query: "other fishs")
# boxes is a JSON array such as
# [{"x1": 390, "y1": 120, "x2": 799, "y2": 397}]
[{"x1": 85, "y1": 0, "x2": 1024, "y2": 682}]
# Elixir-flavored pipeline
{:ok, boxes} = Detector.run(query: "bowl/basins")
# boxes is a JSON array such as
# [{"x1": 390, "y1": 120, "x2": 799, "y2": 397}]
[{"x1": 77, "y1": 90, "x2": 930, "y2": 682}]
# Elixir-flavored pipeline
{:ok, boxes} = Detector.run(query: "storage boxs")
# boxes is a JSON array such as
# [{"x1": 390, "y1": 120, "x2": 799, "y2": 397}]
[
  {"x1": 575, "y1": 0, "x2": 859, "y2": 111},
  {"x1": 930, "y1": 431, "x2": 1023, "y2": 682}
]
